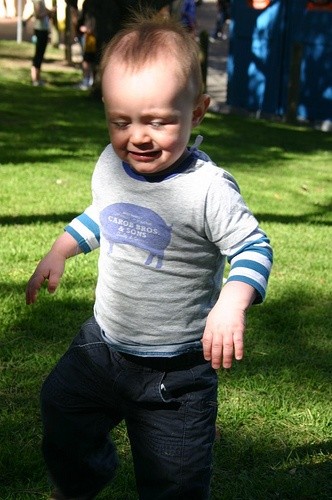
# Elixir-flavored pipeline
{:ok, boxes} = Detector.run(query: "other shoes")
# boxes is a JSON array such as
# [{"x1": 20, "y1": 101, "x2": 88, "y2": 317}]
[
  {"x1": 296, "y1": 115, "x2": 332, "y2": 132},
  {"x1": 47, "y1": 487, "x2": 99, "y2": 500}
]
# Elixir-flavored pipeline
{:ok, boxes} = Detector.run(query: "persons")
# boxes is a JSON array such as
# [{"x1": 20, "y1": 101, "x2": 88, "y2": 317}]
[
  {"x1": 27, "y1": 0, "x2": 332, "y2": 135},
  {"x1": 25, "y1": 16, "x2": 276, "y2": 500}
]
